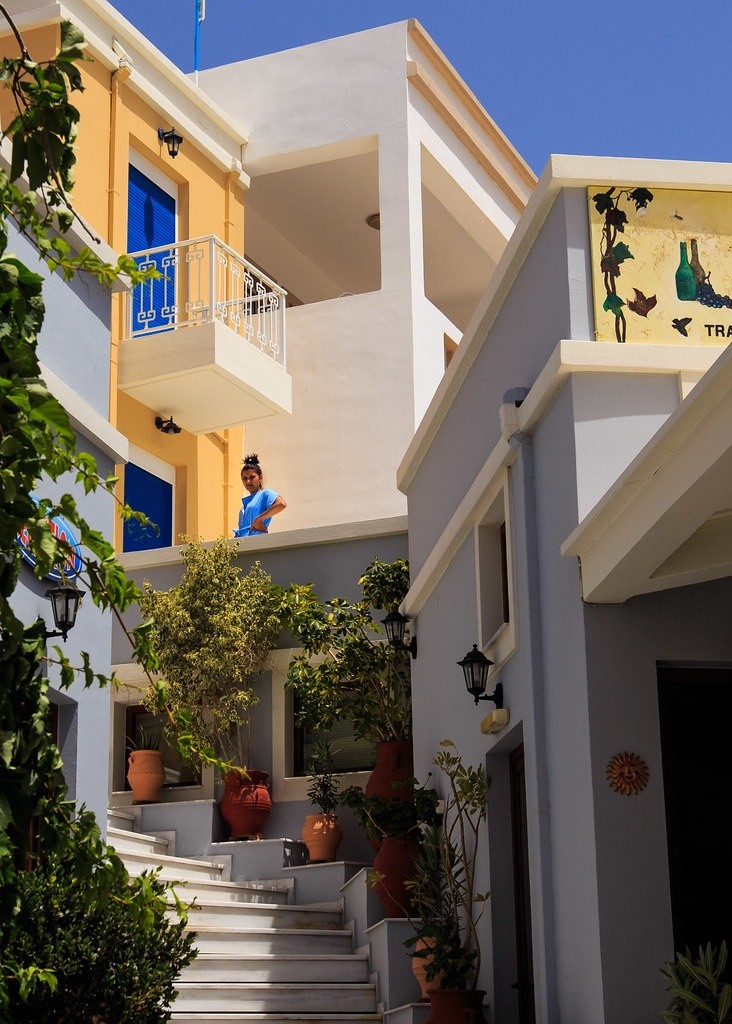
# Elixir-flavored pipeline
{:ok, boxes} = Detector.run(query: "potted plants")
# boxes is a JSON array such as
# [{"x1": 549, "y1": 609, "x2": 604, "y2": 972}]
[
  {"x1": 137, "y1": 537, "x2": 278, "y2": 838},
  {"x1": 295, "y1": 727, "x2": 365, "y2": 859},
  {"x1": 341, "y1": 739, "x2": 502, "y2": 1024},
  {"x1": 115, "y1": 725, "x2": 170, "y2": 805},
  {"x1": 281, "y1": 561, "x2": 415, "y2": 803}
]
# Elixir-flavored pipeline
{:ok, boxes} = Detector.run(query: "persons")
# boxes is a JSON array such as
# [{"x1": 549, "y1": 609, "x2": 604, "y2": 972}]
[{"x1": 233, "y1": 456, "x2": 286, "y2": 538}]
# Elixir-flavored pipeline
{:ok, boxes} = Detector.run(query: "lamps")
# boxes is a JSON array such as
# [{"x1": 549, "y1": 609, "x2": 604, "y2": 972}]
[
  {"x1": 18, "y1": 572, "x2": 86, "y2": 642},
  {"x1": 366, "y1": 213, "x2": 383, "y2": 230},
  {"x1": 456, "y1": 641, "x2": 509, "y2": 709},
  {"x1": 155, "y1": 416, "x2": 182, "y2": 435},
  {"x1": 379, "y1": 604, "x2": 418, "y2": 658},
  {"x1": 157, "y1": 126, "x2": 184, "y2": 160}
]
